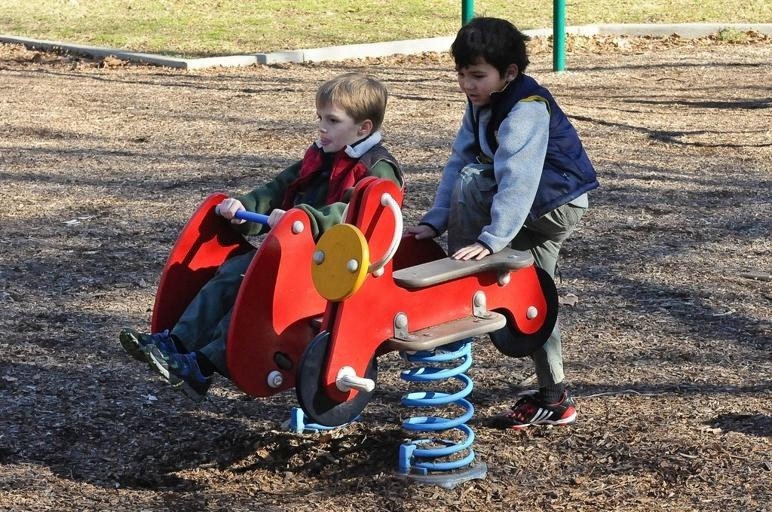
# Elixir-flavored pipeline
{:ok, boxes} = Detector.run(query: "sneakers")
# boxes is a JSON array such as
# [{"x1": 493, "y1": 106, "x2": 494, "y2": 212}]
[
  {"x1": 490, "y1": 391, "x2": 577, "y2": 430},
  {"x1": 120, "y1": 329, "x2": 214, "y2": 402}
]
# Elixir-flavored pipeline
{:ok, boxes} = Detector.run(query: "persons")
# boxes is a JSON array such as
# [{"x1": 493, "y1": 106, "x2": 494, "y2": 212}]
[
  {"x1": 119, "y1": 73, "x2": 407, "y2": 403},
  {"x1": 409, "y1": 17, "x2": 600, "y2": 428}
]
